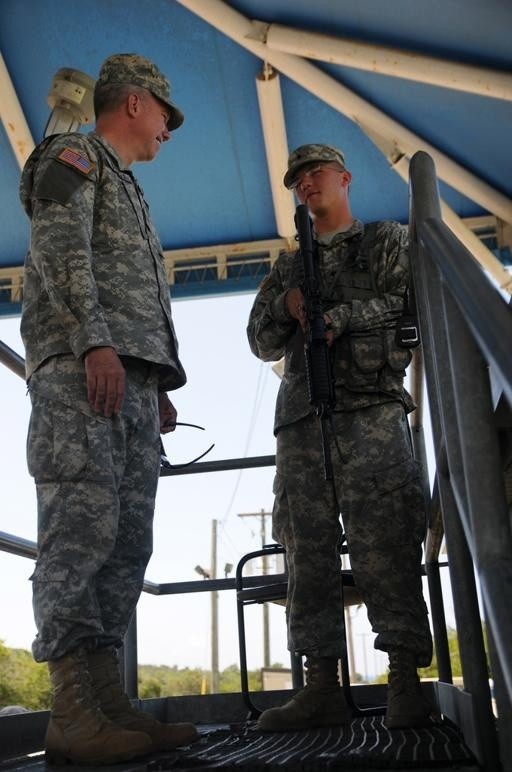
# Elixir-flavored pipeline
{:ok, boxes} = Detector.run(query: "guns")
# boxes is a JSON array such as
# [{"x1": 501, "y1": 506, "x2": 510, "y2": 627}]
[{"x1": 290, "y1": 205, "x2": 338, "y2": 484}]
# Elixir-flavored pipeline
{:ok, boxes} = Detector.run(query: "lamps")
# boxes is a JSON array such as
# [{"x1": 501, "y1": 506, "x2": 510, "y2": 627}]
[{"x1": 38, "y1": 68, "x2": 97, "y2": 138}]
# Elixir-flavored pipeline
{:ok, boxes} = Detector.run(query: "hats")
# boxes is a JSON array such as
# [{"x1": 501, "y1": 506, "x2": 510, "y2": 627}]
[
  {"x1": 284, "y1": 143, "x2": 346, "y2": 190},
  {"x1": 96, "y1": 52, "x2": 186, "y2": 132}
]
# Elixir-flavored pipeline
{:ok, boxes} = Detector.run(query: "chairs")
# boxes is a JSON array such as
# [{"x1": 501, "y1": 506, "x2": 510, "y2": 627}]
[{"x1": 235, "y1": 535, "x2": 392, "y2": 719}]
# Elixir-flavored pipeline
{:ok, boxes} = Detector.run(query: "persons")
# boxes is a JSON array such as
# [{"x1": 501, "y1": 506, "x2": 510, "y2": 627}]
[
  {"x1": 244, "y1": 143, "x2": 446, "y2": 732},
  {"x1": 19, "y1": 51, "x2": 201, "y2": 766}
]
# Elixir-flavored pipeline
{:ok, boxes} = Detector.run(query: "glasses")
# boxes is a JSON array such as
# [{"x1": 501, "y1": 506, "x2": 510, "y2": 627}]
[{"x1": 284, "y1": 163, "x2": 344, "y2": 193}]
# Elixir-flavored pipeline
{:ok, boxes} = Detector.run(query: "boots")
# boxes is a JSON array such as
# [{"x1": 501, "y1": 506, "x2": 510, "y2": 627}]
[
  {"x1": 386, "y1": 644, "x2": 429, "y2": 729},
  {"x1": 254, "y1": 660, "x2": 352, "y2": 734},
  {"x1": 88, "y1": 644, "x2": 199, "y2": 750},
  {"x1": 43, "y1": 643, "x2": 152, "y2": 767}
]
